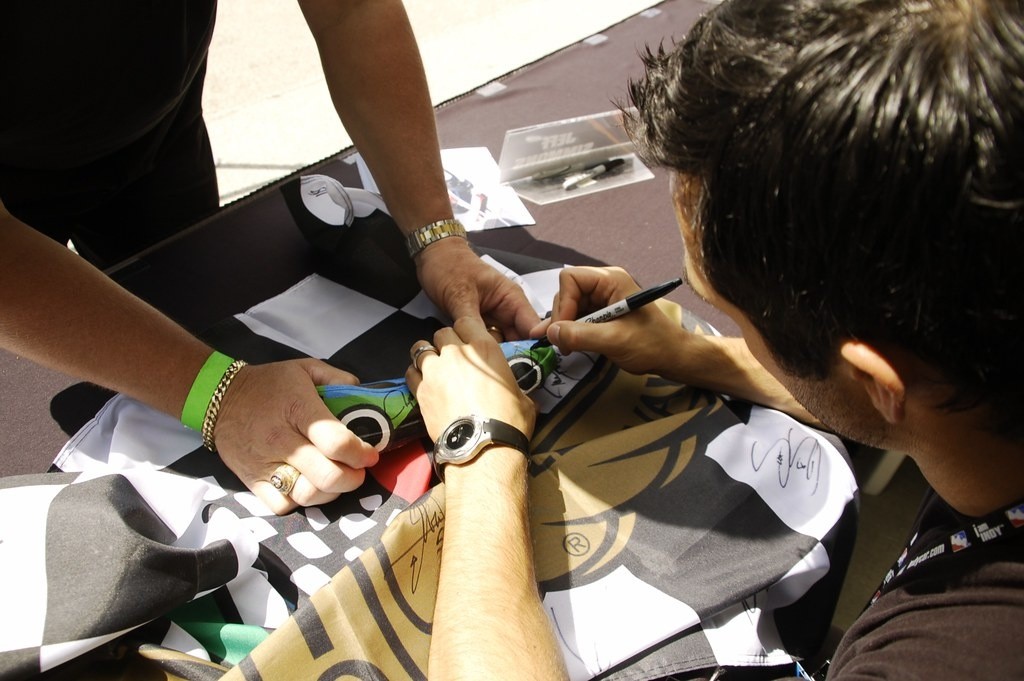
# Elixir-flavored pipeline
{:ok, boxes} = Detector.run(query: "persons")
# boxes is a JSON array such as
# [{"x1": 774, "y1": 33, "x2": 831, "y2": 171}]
[
  {"x1": 0, "y1": 0, "x2": 541, "y2": 520},
  {"x1": 405, "y1": 0, "x2": 1024, "y2": 681}
]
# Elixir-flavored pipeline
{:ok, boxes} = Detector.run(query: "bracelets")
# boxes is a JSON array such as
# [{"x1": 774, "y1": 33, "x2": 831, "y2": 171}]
[
  {"x1": 180, "y1": 350, "x2": 248, "y2": 450},
  {"x1": 405, "y1": 219, "x2": 466, "y2": 258}
]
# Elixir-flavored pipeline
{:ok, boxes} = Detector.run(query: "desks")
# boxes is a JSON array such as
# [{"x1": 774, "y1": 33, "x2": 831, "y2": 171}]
[{"x1": 0, "y1": 0, "x2": 932, "y2": 681}]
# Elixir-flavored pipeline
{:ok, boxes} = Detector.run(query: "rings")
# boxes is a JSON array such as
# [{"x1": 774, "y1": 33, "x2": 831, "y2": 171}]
[
  {"x1": 269, "y1": 464, "x2": 301, "y2": 496},
  {"x1": 413, "y1": 345, "x2": 440, "y2": 370},
  {"x1": 486, "y1": 326, "x2": 503, "y2": 335}
]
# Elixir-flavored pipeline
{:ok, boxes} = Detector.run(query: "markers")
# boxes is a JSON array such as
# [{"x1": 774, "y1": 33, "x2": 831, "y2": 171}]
[
  {"x1": 530, "y1": 278, "x2": 684, "y2": 351},
  {"x1": 562, "y1": 158, "x2": 626, "y2": 190}
]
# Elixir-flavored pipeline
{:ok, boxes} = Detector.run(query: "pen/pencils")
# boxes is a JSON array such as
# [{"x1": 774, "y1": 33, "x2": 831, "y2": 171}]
[{"x1": 530, "y1": 158, "x2": 608, "y2": 181}]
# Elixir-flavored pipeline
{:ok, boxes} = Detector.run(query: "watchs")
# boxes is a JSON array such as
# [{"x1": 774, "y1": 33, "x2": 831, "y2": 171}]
[{"x1": 433, "y1": 412, "x2": 532, "y2": 485}]
